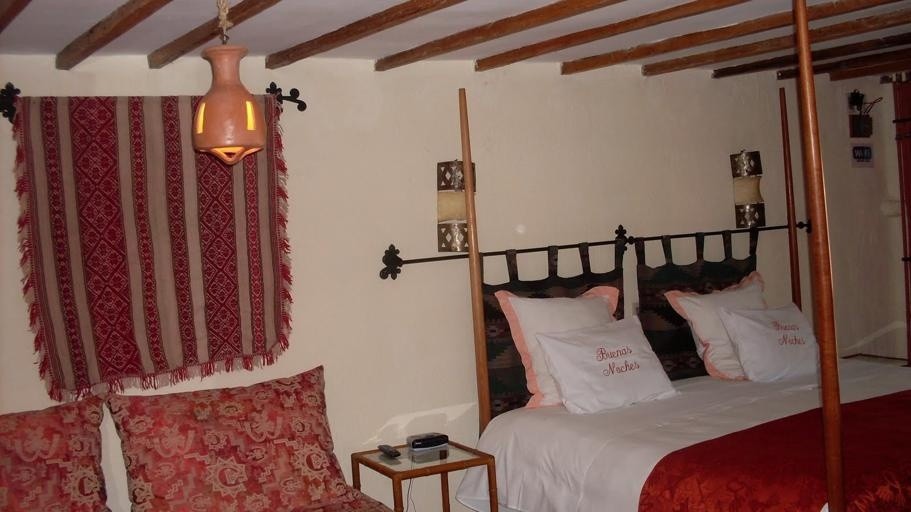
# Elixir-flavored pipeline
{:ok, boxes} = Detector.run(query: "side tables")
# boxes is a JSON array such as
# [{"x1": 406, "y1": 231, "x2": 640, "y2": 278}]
[{"x1": 350, "y1": 440, "x2": 498, "y2": 512}]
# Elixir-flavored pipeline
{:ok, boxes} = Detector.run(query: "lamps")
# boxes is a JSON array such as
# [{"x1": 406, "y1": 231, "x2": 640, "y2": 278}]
[
  {"x1": 191, "y1": 0, "x2": 266, "y2": 167},
  {"x1": 435, "y1": 157, "x2": 478, "y2": 253},
  {"x1": 729, "y1": 151, "x2": 768, "y2": 229}
]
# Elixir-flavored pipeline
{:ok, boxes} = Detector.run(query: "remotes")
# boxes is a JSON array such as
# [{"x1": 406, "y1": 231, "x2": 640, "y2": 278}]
[{"x1": 378, "y1": 443, "x2": 400, "y2": 458}]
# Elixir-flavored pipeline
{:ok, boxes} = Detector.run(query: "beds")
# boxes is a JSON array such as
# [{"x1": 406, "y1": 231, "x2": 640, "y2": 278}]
[{"x1": 464, "y1": 220, "x2": 910, "y2": 511}]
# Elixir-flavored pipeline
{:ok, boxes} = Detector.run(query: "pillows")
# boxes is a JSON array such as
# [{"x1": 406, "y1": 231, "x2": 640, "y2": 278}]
[
  {"x1": 663, "y1": 270, "x2": 821, "y2": 384},
  {"x1": 105, "y1": 365, "x2": 362, "y2": 511},
  {"x1": 492, "y1": 286, "x2": 683, "y2": 415},
  {"x1": 1, "y1": 396, "x2": 112, "y2": 512}
]
